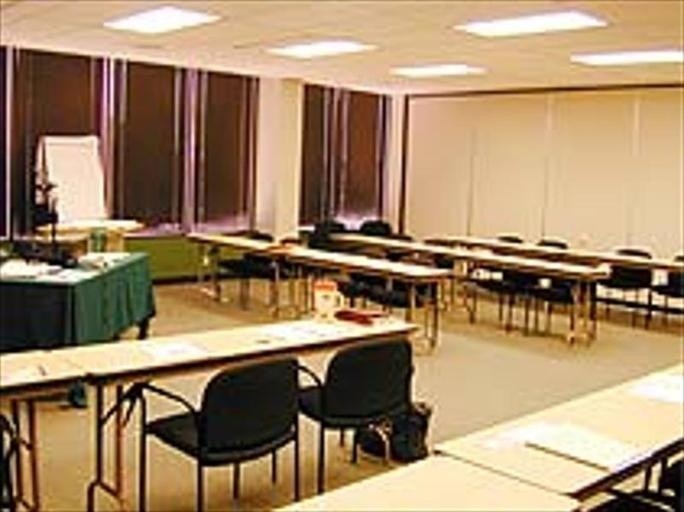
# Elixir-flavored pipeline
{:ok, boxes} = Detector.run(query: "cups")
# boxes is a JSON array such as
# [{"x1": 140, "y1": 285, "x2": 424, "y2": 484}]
[{"x1": 313, "y1": 280, "x2": 345, "y2": 323}]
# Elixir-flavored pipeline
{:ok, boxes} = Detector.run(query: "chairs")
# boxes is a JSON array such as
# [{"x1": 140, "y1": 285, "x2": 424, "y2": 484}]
[
  {"x1": 189, "y1": 217, "x2": 684, "y2": 350},
  {"x1": 578, "y1": 439, "x2": 684, "y2": 510}
]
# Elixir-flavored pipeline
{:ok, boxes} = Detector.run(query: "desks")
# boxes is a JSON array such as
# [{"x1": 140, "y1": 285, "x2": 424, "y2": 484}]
[
  {"x1": 276, "y1": 454, "x2": 578, "y2": 510},
  {"x1": 425, "y1": 361, "x2": 684, "y2": 505},
  {"x1": 0, "y1": 232, "x2": 420, "y2": 511}
]
